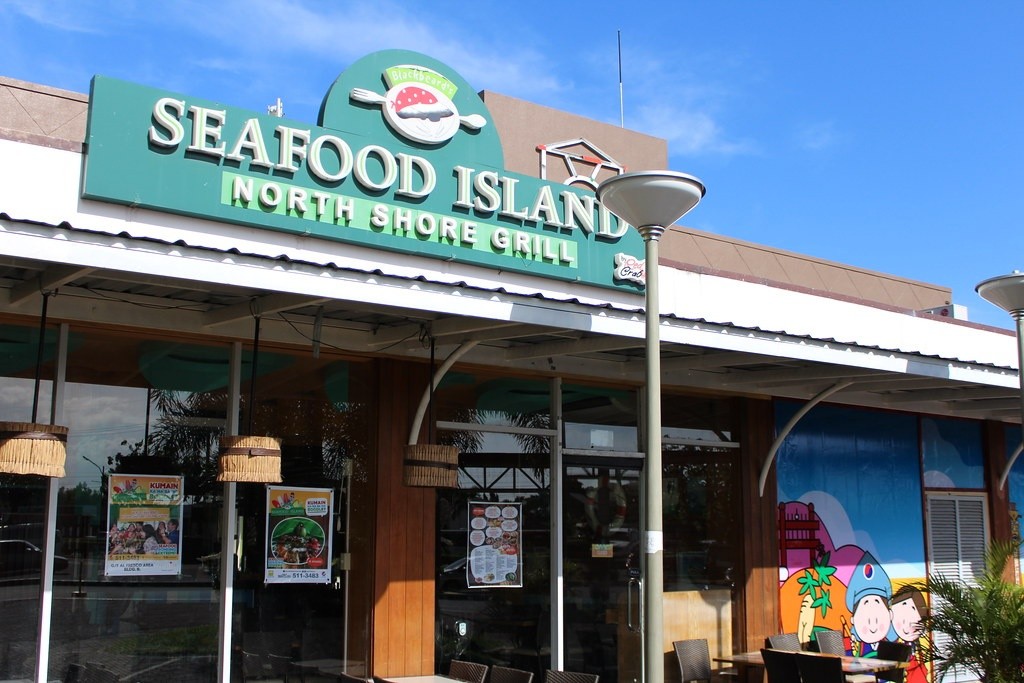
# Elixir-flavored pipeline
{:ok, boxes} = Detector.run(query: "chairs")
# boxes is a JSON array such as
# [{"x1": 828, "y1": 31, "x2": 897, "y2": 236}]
[
  {"x1": 672, "y1": 630, "x2": 912, "y2": 683},
  {"x1": 341, "y1": 660, "x2": 599, "y2": 683}
]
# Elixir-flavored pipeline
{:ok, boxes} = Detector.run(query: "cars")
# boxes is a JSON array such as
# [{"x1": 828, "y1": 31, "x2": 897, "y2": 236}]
[{"x1": 0, "y1": 540, "x2": 69, "y2": 572}]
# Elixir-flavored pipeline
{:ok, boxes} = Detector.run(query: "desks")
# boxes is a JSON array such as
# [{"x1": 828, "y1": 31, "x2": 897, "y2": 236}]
[{"x1": 713, "y1": 649, "x2": 910, "y2": 683}]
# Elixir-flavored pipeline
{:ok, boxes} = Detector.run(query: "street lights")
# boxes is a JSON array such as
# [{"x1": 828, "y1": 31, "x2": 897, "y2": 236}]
[
  {"x1": 595, "y1": 169, "x2": 707, "y2": 683},
  {"x1": 975, "y1": 270, "x2": 1024, "y2": 418}
]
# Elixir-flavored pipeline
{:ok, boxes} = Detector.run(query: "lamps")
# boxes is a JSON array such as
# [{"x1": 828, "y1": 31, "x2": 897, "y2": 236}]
[
  {"x1": 0, "y1": 293, "x2": 69, "y2": 479},
  {"x1": 402, "y1": 322, "x2": 461, "y2": 488},
  {"x1": 217, "y1": 316, "x2": 282, "y2": 483}
]
